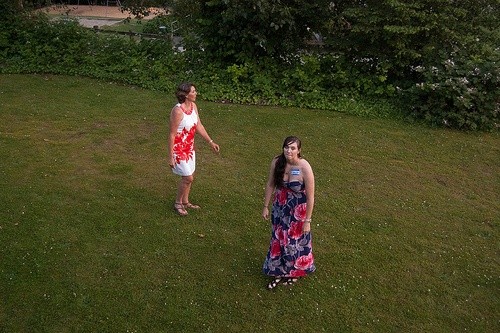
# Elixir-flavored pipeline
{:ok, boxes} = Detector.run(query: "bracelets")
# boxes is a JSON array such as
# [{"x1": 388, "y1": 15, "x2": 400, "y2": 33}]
[
  {"x1": 208, "y1": 140, "x2": 213, "y2": 144},
  {"x1": 264, "y1": 206, "x2": 268, "y2": 207}
]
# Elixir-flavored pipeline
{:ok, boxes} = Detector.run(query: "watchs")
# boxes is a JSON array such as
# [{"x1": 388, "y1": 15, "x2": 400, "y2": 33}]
[{"x1": 304, "y1": 218, "x2": 312, "y2": 223}]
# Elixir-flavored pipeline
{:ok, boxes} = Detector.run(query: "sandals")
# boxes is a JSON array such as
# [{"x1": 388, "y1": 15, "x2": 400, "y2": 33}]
[
  {"x1": 182, "y1": 202, "x2": 201, "y2": 209},
  {"x1": 282, "y1": 277, "x2": 299, "y2": 286},
  {"x1": 266, "y1": 277, "x2": 283, "y2": 291},
  {"x1": 174, "y1": 202, "x2": 189, "y2": 216}
]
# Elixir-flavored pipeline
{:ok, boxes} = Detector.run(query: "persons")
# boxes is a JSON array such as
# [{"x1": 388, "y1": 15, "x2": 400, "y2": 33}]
[
  {"x1": 169, "y1": 83, "x2": 220, "y2": 215},
  {"x1": 264, "y1": 135, "x2": 316, "y2": 289}
]
